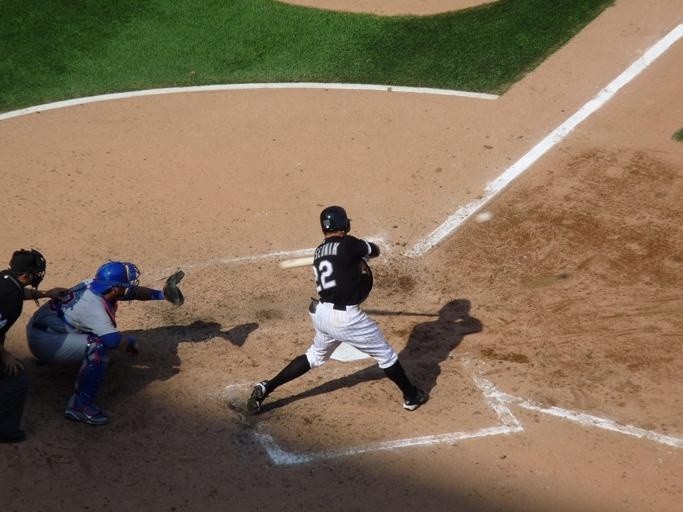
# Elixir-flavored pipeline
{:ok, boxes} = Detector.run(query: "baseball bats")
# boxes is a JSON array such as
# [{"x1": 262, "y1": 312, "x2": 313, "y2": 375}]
[{"x1": 277, "y1": 258, "x2": 315, "y2": 270}]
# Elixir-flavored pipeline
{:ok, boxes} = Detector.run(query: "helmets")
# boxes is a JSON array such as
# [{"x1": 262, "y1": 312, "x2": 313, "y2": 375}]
[
  {"x1": 320, "y1": 206, "x2": 350, "y2": 233},
  {"x1": 90, "y1": 261, "x2": 141, "y2": 298},
  {"x1": 8, "y1": 249, "x2": 46, "y2": 287}
]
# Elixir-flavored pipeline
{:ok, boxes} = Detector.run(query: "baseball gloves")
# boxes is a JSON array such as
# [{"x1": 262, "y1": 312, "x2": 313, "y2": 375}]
[{"x1": 163, "y1": 270, "x2": 185, "y2": 306}]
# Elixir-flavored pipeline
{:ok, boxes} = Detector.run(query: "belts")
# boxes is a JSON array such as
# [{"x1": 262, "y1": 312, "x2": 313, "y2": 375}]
[{"x1": 333, "y1": 304, "x2": 346, "y2": 312}]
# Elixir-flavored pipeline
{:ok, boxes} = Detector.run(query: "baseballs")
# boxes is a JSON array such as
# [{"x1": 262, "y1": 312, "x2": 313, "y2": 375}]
[{"x1": 478, "y1": 212, "x2": 492, "y2": 222}]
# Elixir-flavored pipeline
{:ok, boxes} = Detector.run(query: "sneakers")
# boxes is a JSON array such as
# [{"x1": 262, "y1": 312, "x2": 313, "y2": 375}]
[
  {"x1": 246, "y1": 380, "x2": 270, "y2": 416},
  {"x1": 65, "y1": 397, "x2": 110, "y2": 425},
  {"x1": 403, "y1": 391, "x2": 429, "y2": 411},
  {"x1": 0, "y1": 429, "x2": 26, "y2": 443}
]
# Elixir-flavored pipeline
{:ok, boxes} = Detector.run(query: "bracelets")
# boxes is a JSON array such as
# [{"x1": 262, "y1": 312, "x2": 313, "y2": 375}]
[
  {"x1": 126, "y1": 335, "x2": 135, "y2": 346},
  {"x1": 41, "y1": 290, "x2": 46, "y2": 297},
  {"x1": 151, "y1": 289, "x2": 164, "y2": 301}
]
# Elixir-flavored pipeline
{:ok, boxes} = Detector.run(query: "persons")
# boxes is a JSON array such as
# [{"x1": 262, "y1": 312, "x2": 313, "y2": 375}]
[
  {"x1": 24, "y1": 260, "x2": 183, "y2": 425},
  {"x1": 245, "y1": 202, "x2": 430, "y2": 414},
  {"x1": 0, "y1": 248, "x2": 70, "y2": 443}
]
